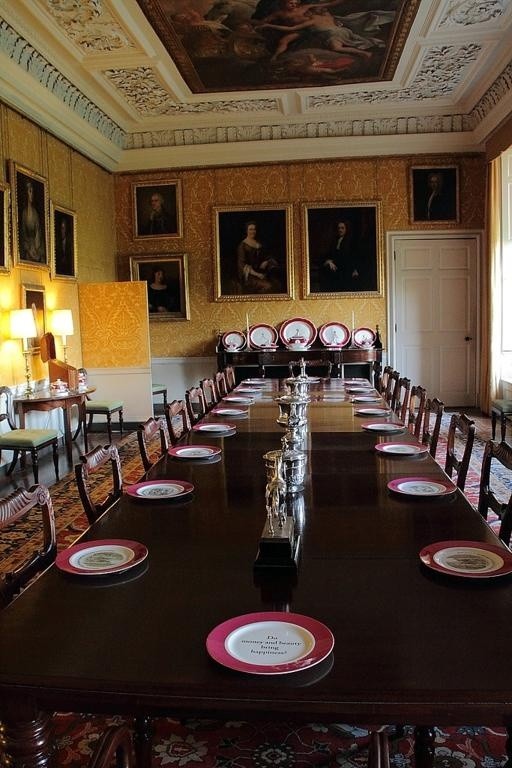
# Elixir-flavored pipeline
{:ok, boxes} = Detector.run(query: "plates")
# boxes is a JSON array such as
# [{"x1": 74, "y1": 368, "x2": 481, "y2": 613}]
[
  {"x1": 167, "y1": 445, "x2": 221, "y2": 460},
  {"x1": 354, "y1": 327, "x2": 375, "y2": 348},
  {"x1": 216, "y1": 414, "x2": 247, "y2": 420},
  {"x1": 355, "y1": 406, "x2": 391, "y2": 416},
  {"x1": 168, "y1": 455, "x2": 221, "y2": 465},
  {"x1": 55, "y1": 538, "x2": 149, "y2": 575},
  {"x1": 206, "y1": 611, "x2": 336, "y2": 675},
  {"x1": 278, "y1": 317, "x2": 317, "y2": 348},
  {"x1": 344, "y1": 380, "x2": 368, "y2": 385},
  {"x1": 193, "y1": 422, "x2": 236, "y2": 433},
  {"x1": 353, "y1": 395, "x2": 382, "y2": 402},
  {"x1": 72, "y1": 558, "x2": 149, "y2": 589},
  {"x1": 130, "y1": 493, "x2": 195, "y2": 508},
  {"x1": 346, "y1": 386, "x2": 376, "y2": 393},
  {"x1": 194, "y1": 429, "x2": 236, "y2": 438},
  {"x1": 386, "y1": 476, "x2": 458, "y2": 497},
  {"x1": 221, "y1": 330, "x2": 247, "y2": 350},
  {"x1": 211, "y1": 409, "x2": 248, "y2": 416},
  {"x1": 242, "y1": 381, "x2": 266, "y2": 385},
  {"x1": 249, "y1": 323, "x2": 279, "y2": 350},
  {"x1": 318, "y1": 321, "x2": 350, "y2": 346},
  {"x1": 374, "y1": 442, "x2": 428, "y2": 456},
  {"x1": 361, "y1": 421, "x2": 405, "y2": 433},
  {"x1": 238, "y1": 388, "x2": 259, "y2": 393},
  {"x1": 127, "y1": 479, "x2": 195, "y2": 500},
  {"x1": 222, "y1": 397, "x2": 254, "y2": 403},
  {"x1": 224, "y1": 400, "x2": 255, "y2": 406},
  {"x1": 418, "y1": 539, "x2": 511, "y2": 578},
  {"x1": 294, "y1": 651, "x2": 335, "y2": 688}
]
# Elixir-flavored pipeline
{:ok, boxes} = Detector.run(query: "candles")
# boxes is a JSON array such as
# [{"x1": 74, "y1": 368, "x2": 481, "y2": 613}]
[
  {"x1": 351, "y1": 311, "x2": 355, "y2": 331},
  {"x1": 245, "y1": 312, "x2": 250, "y2": 332}
]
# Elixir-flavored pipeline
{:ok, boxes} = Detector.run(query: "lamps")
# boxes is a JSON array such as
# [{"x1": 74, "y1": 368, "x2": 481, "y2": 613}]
[
  {"x1": 9, "y1": 309, "x2": 39, "y2": 399},
  {"x1": 52, "y1": 308, "x2": 75, "y2": 364}
]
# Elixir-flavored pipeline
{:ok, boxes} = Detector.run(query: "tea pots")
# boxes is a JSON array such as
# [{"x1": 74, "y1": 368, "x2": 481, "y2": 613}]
[{"x1": 50, "y1": 378, "x2": 68, "y2": 396}]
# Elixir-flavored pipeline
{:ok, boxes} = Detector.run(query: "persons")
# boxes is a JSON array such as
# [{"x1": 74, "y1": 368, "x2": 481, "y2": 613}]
[
  {"x1": 237, "y1": 217, "x2": 281, "y2": 293},
  {"x1": 170, "y1": 0, "x2": 373, "y2": 76},
  {"x1": 58, "y1": 218, "x2": 70, "y2": 275},
  {"x1": 19, "y1": 180, "x2": 44, "y2": 262},
  {"x1": 144, "y1": 265, "x2": 180, "y2": 312},
  {"x1": 315, "y1": 222, "x2": 371, "y2": 288},
  {"x1": 138, "y1": 192, "x2": 177, "y2": 232},
  {"x1": 418, "y1": 174, "x2": 456, "y2": 220}
]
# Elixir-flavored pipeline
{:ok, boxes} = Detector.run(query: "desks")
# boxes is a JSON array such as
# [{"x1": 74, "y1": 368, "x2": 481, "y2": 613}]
[{"x1": 214, "y1": 323, "x2": 386, "y2": 384}]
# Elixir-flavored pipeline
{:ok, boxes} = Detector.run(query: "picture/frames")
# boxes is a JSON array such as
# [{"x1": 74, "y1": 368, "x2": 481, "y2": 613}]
[
  {"x1": 20, "y1": 282, "x2": 47, "y2": 357},
  {"x1": 406, "y1": 164, "x2": 461, "y2": 226},
  {"x1": 128, "y1": 252, "x2": 191, "y2": 323},
  {"x1": 49, "y1": 199, "x2": 79, "y2": 283},
  {"x1": 210, "y1": 202, "x2": 295, "y2": 302},
  {"x1": 131, "y1": 177, "x2": 183, "y2": 243},
  {"x1": 0, "y1": 181, "x2": 12, "y2": 273},
  {"x1": 299, "y1": 199, "x2": 385, "y2": 300},
  {"x1": 5, "y1": 158, "x2": 52, "y2": 272}
]
